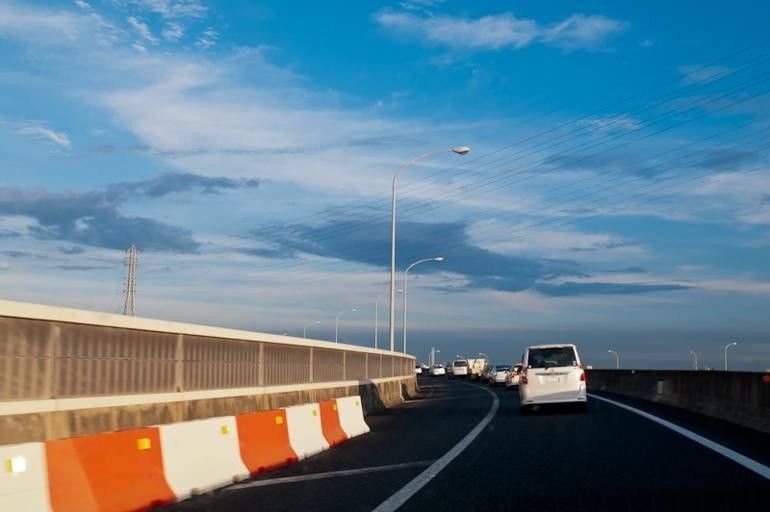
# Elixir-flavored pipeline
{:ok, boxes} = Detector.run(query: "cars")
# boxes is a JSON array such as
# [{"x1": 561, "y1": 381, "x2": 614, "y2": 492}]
[
  {"x1": 544, "y1": 360, "x2": 557, "y2": 365},
  {"x1": 413, "y1": 359, "x2": 514, "y2": 386},
  {"x1": 505, "y1": 362, "x2": 522, "y2": 389}
]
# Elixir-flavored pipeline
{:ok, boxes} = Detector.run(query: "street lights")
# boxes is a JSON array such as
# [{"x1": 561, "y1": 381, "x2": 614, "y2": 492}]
[
  {"x1": 303, "y1": 320, "x2": 321, "y2": 339},
  {"x1": 335, "y1": 308, "x2": 358, "y2": 344},
  {"x1": 479, "y1": 353, "x2": 488, "y2": 367},
  {"x1": 725, "y1": 342, "x2": 737, "y2": 372},
  {"x1": 689, "y1": 350, "x2": 698, "y2": 370},
  {"x1": 375, "y1": 290, "x2": 403, "y2": 349},
  {"x1": 390, "y1": 146, "x2": 468, "y2": 352},
  {"x1": 429, "y1": 349, "x2": 440, "y2": 370},
  {"x1": 403, "y1": 256, "x2": 445, "y2": 355},
  {"x1": 609, "y1": 349, "x2": 619, "y2": 369},
  {"x1": 281, "y1": 332, "x2": 287, "y2": 336},
  {"x1": 456, "y1": 355, "x2": 465, "y2": 360}
]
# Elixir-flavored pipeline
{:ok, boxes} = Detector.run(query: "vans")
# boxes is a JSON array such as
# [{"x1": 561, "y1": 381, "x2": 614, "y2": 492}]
[{"x1": 514, "y1": 343, "x2": 594, "y2": 414}]
